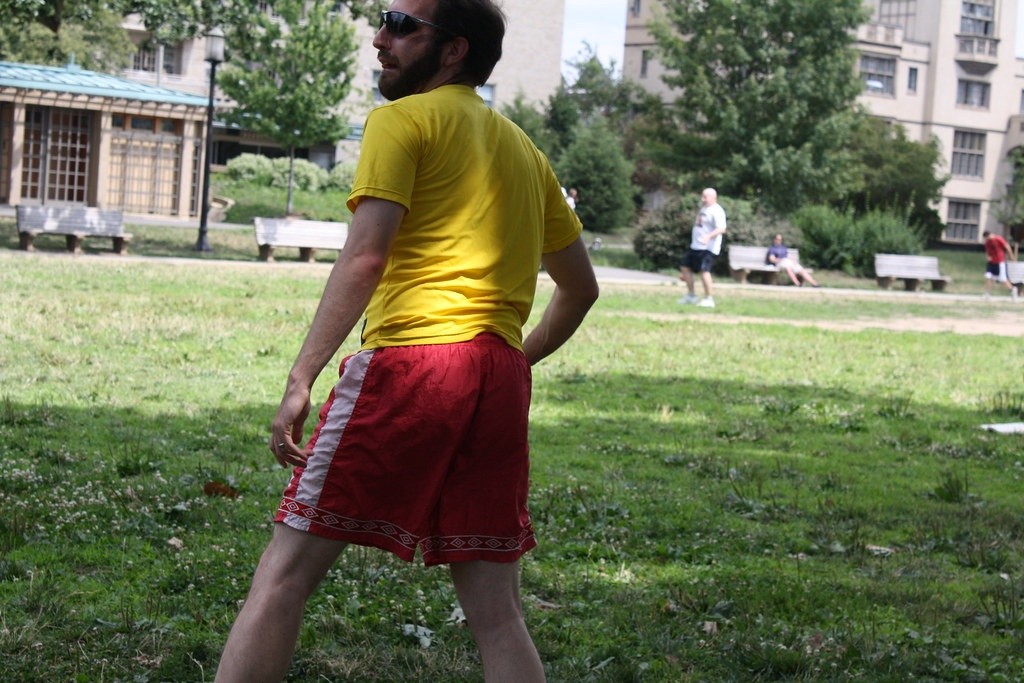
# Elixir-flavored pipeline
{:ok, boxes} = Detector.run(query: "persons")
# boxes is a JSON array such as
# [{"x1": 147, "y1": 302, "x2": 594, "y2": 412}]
[
  {"x1": 557, "y1": 181, "x2": 576, "y2": 209},
  {"x1": 765, "y1": 233, "x2": 822, "y2": 287},
  {"x1": 679, "y1": 188, "x2": 726, "y2": 308},
  {"x1": 982, "y1": 230, "x2": 1018, "y2": 301},
  {"x1": 211, "y1": 0, "x2": 599, "y2": 683}
]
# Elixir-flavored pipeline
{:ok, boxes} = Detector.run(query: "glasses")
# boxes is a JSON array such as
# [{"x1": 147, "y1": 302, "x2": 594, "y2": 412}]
[{"x1": 379, "y1": 10, "x2": 455, "y2": 36}]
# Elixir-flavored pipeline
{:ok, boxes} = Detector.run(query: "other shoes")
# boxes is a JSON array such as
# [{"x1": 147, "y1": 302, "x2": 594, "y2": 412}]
[
  {"x1": 697, "y1": 298, "x2": 715, "y2": 306},
  {"x1": 679, "y1": 295, "x2": 697, "y2": 303},
  {"x1": 1010, "y1": 288, "x2": 1018, "y2": 298}
]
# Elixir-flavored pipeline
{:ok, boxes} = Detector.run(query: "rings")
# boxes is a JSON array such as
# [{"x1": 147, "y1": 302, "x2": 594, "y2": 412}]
[{"x1": 278, "y1": 443, "x2": 284, "y2": 446}]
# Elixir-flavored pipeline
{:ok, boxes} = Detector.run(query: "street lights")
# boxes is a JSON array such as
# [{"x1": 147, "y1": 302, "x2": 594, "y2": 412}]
[{"x1": 190, "y1": 24, "x2": 227, "y2": 252}]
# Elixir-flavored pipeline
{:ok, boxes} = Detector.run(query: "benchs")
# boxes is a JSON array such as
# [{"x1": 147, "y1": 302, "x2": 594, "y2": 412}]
[
  {"x1": 255, "y1": 217, "x2": 350, "y2": 264},
  {"x1": 875, "y1": 254, "x2": 951, "y2": 291},
  {"x1": 997, "y1": 260, "x2": 1024, "y2": 296},
  {"x1": 728, "y1": 245, "x2": 813, "y2": 287},
  {"x1": 14, "y1": 206, "x2": 135, "y2": 253}
]
